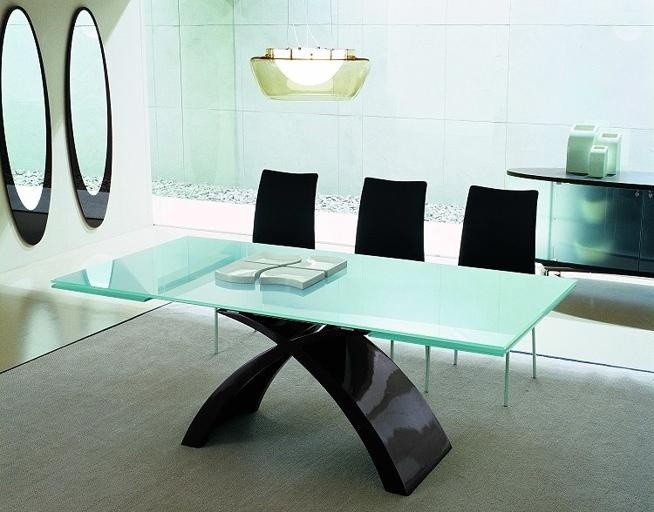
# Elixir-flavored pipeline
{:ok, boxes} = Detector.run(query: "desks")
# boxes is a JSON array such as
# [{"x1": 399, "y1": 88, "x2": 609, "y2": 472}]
[
  {"x1": 510, "y1": 169, "x2": 654, "y2": 281},
  {"x1": 47, "y1": 233, "x2": 581, "y2": 497}
]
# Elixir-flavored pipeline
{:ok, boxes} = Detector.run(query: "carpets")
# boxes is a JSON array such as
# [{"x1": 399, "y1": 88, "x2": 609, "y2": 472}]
[
  {"x1": 0, "y1": 300, "x2": 653, "y2": 511},
  {"x1": 552, "y1": 279, "x2": 654, "y2": 332}
]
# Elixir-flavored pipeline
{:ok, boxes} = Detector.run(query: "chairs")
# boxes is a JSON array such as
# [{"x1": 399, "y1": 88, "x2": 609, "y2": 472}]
[
  {"x1": 213, "y1": 169, "x2": 317, "y2": 355},
  {"x1": 421, "y1": 184, "x2": 540, "y2": 408},
  {"x1": 353, "y1": 175, "x2": 427, "y2": 363}
]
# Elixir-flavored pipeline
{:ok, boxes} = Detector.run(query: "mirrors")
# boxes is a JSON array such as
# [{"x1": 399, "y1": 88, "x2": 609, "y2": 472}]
[
  {"x1": 65, "y1": 6, "x2": 112, "y2": 231},
  {"x1": 0, "y1": 5, "x2": 51, "y2": 247}
]
